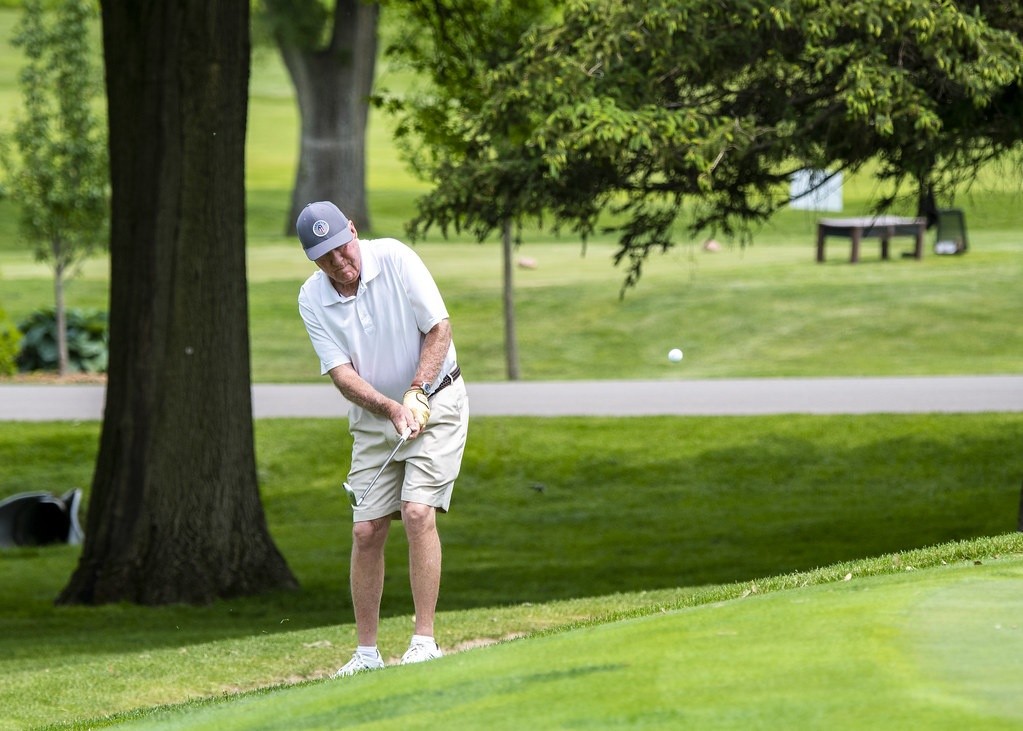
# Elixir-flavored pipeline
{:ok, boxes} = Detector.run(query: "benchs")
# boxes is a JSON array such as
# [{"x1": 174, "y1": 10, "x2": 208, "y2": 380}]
[{"x1": 816, "y1": 215, "x2": 925, "y2": 262}]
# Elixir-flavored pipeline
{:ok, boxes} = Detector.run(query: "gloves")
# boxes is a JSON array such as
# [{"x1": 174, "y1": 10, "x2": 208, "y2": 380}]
[{"x1": 403, "y1": 389, "x2": 430, "y2": 427}]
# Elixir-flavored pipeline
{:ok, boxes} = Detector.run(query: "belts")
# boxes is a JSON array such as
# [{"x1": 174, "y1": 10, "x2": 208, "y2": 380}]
[{"x1": 429, "y1": 365, "x2": 461, "y2": 396}]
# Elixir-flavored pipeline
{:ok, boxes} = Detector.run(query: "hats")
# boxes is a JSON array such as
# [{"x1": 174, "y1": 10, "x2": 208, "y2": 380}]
[{"x1": 296, "y1": 201, "x2": 353, "y2": 261}]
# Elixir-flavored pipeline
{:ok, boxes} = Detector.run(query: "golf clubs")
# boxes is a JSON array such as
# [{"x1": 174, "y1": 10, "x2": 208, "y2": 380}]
[{"x1": 342, "y1": 427, "x2": 412, "y2": 508}]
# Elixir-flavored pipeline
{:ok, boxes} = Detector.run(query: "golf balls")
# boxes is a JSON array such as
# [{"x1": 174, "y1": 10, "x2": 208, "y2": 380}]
[{"x1": 668, "y1": 348, "x2": 683, "y2": 363}]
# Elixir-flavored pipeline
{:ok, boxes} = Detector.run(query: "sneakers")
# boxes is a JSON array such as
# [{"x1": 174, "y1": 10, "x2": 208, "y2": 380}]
[
  {"x1": 399, "y1": 642, "x2": 443, "y2": 664},
  {"x1": 337, "y1": 649, "x2": 384, "y2": 675}
]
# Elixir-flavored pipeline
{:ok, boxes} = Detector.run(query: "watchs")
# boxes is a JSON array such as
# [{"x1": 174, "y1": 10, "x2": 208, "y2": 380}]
[{"x1": 411, "y1": 381, "x2": 431, "y2": 394}]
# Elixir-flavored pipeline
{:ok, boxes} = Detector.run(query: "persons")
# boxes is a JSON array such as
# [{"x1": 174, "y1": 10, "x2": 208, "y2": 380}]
[{"x1": 296, "y1": 200, "x2": 468, "y2": 676}]
